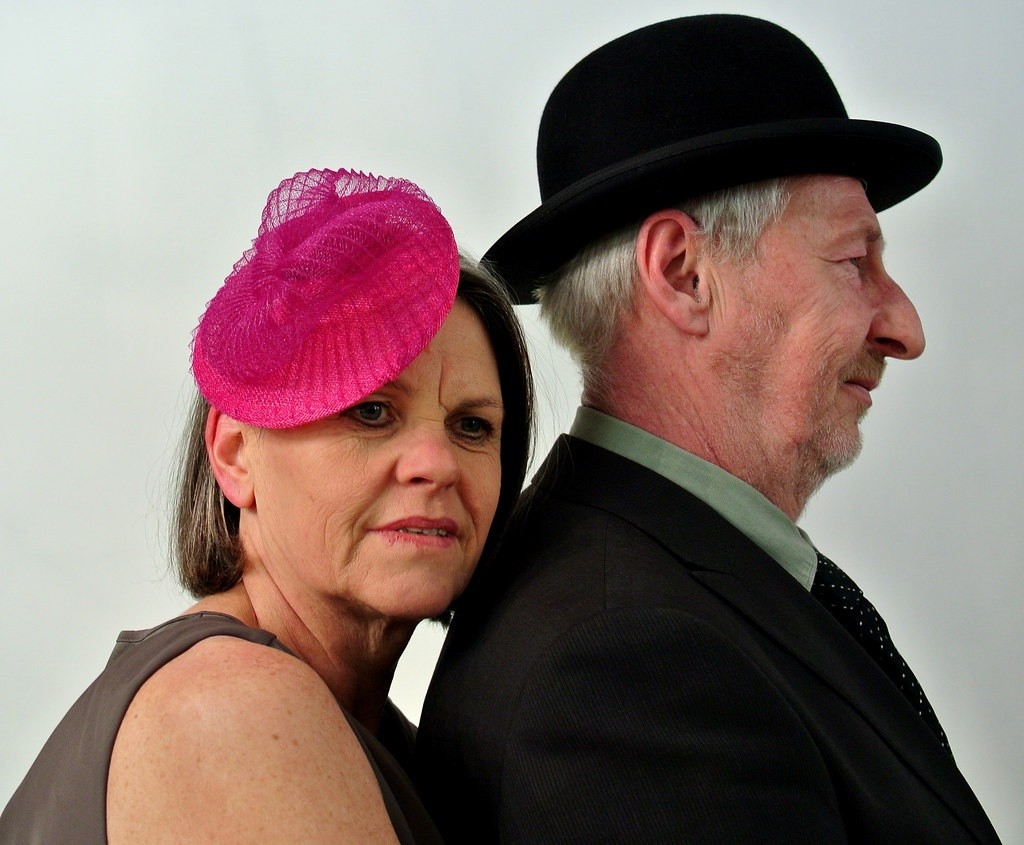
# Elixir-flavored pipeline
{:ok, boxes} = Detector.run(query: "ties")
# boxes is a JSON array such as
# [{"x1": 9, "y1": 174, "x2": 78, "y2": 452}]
[{"x1": 810, "y1": 547, "x2": 955, "y2": 766}]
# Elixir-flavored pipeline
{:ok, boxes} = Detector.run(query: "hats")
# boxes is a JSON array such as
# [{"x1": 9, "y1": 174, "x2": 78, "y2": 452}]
[{"x1": 479, "y1": 13, "x2": 944, "y2": 303}]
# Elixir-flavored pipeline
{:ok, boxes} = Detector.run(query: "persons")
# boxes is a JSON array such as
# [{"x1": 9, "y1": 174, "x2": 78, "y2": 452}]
[
  {"x1": 3, "y1": 170, "x2": 535, "y2": 845},
  {"x1": 414, "y1": 13, "x2": 1001, "y2": 845}
]
